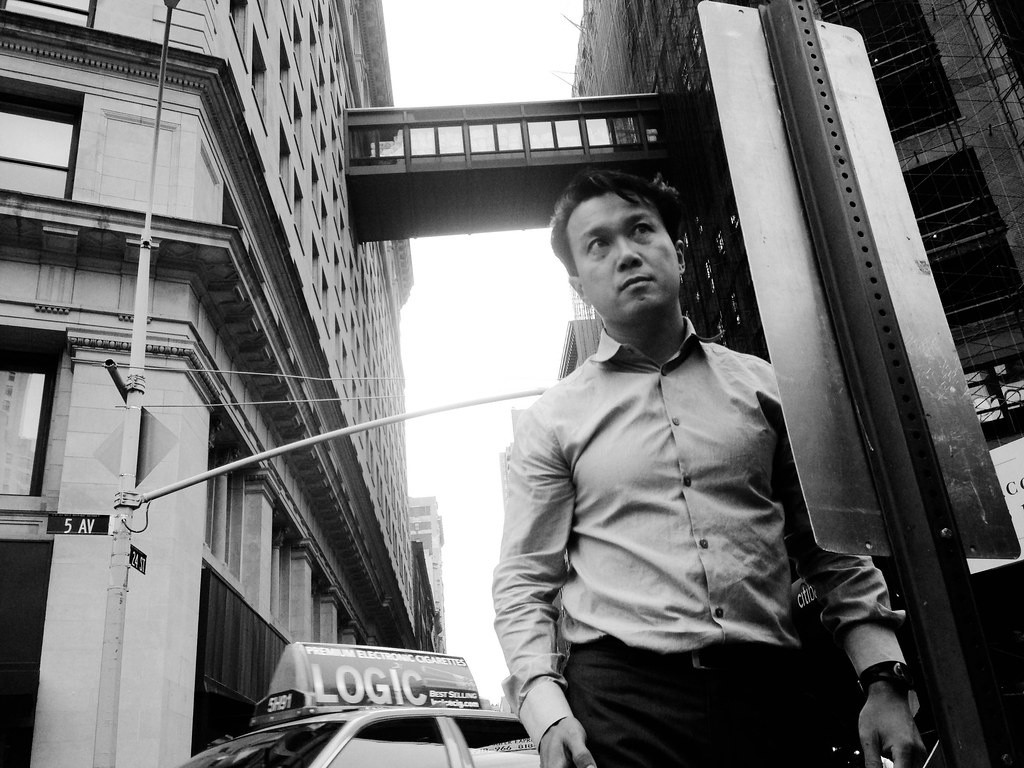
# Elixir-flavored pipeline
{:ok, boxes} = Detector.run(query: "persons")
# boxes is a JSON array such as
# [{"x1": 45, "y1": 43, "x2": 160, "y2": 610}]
[{"x1": 493, "y1": 169, "x2": 927, "y2": 768}]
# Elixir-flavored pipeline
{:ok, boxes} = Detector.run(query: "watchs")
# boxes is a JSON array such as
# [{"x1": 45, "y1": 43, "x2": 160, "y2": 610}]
[{"x1": 861, "y1": 662, "x2": 914, "y2": 699}]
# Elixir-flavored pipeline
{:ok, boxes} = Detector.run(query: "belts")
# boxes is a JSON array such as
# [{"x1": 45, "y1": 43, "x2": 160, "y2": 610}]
[{"x1": 666, "y1": 647, "x2": 743, "y2": 669}]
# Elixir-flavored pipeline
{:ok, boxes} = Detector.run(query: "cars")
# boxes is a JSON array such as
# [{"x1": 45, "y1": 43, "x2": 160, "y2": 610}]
[{"x1": 176, "y1": 643, "x2": 541, "y2": 767}]
